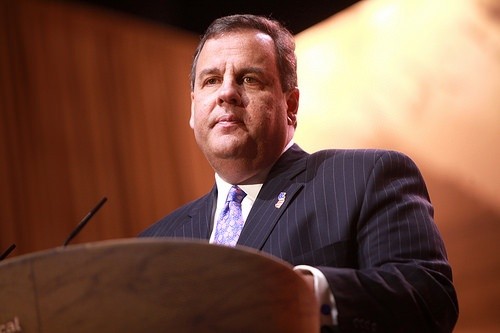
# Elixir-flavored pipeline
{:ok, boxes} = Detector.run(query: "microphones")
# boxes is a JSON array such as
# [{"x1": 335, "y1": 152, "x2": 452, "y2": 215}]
[
  {"x1": 64, "y1": 197, "x2": 107, "y2": 246},
  {"x1": 0, "y1": 243, "x2": 16, "y2": 261}
]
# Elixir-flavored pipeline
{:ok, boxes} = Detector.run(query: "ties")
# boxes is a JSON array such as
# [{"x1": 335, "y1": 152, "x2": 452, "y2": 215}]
[{"x1": 213, "y1": 186, "x2": 248, "y2": 249}]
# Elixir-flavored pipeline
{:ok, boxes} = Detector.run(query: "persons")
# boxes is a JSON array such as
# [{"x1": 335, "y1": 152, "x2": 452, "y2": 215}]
[{"x1": 132, "y1": 12, "x2": 460, "y2": 332}]
